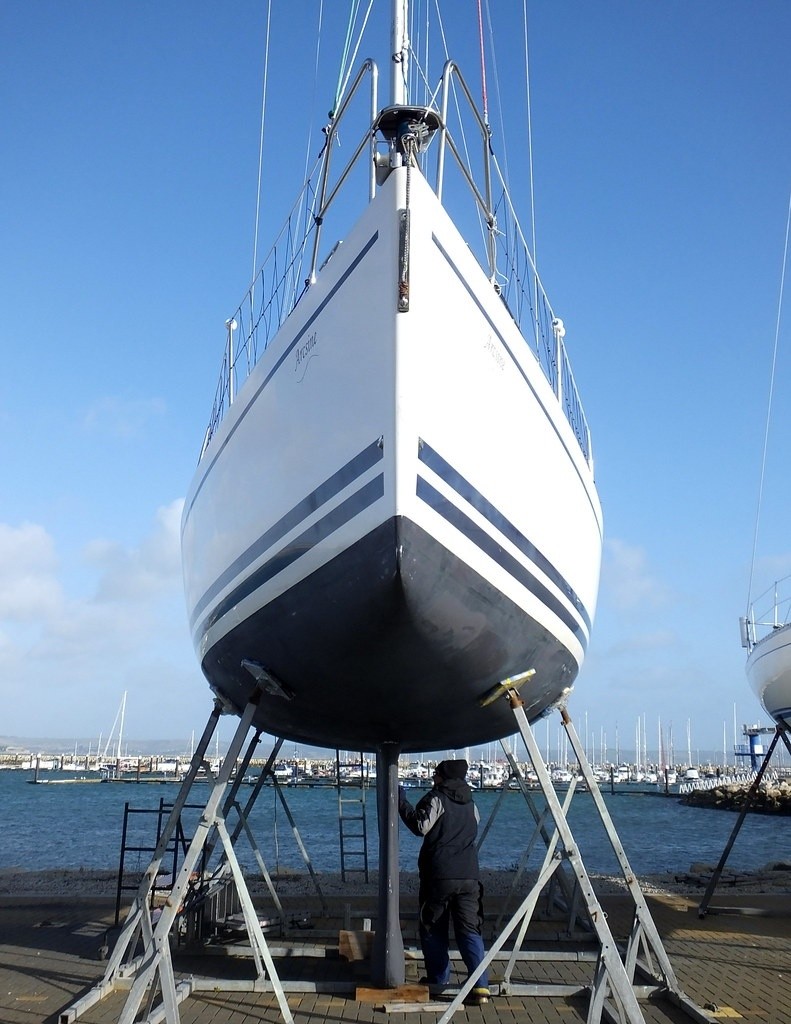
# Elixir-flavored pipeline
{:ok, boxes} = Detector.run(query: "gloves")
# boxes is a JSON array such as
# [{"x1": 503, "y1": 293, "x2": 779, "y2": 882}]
[{"x1": 398, "y1": 785, "x2": 407, "y2": 804}]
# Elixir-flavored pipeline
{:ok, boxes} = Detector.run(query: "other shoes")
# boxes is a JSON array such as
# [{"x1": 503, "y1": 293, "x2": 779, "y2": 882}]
[
  {"x1": 419, "y1": 977, "x2": 427, "y2": 985},
  {"x1": 461, "y1": 979, "x2": 490, "y2": 1004}
]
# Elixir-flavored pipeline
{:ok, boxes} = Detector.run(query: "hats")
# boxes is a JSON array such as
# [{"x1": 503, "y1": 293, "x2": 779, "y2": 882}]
[{"x1": 435, "y1": 760, "x2": 468, "y2": 779}]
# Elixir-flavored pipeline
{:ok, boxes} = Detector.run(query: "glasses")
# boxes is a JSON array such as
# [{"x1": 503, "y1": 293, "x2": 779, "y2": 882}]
[{"x1": 433, "y1": 772, "x2": 437, "y2": 775}]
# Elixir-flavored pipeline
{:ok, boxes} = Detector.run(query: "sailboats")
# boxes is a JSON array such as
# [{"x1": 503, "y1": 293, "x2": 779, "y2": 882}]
[
  {"x1": 182, "y1": 0, "x2": 603, "y2": 755},
  {"x1": 738, "y1": 200, "x2": 791, "y2": 736},
  {"x1": 22, "y1": 692, "x2": 791, "y2": 789}
]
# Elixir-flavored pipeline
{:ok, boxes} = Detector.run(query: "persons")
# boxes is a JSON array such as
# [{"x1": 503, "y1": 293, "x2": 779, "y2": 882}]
[{"x1": 397, "y1": 759, "x2": 491, "y2": 1005}]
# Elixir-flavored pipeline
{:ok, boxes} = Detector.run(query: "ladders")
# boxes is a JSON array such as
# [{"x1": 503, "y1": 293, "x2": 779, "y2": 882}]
[
  {"x1": 98, "y1": 797, "x2": 209, "y2": 961},
  {"x1": 334, "y1": 748, "x2": 369, "y2": 884}
]
